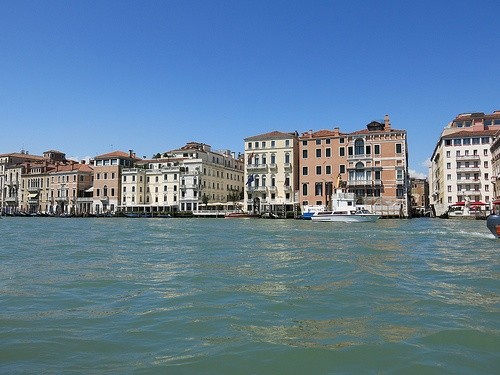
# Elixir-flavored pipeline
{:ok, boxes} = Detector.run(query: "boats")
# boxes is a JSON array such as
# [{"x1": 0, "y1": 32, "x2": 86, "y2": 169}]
[
  {"x1": 310, "y1": 206, "x2": 380, "y2": 222},
  {"x1": 487, "y1": 214, "x2": 500, "y2": 239}
]
